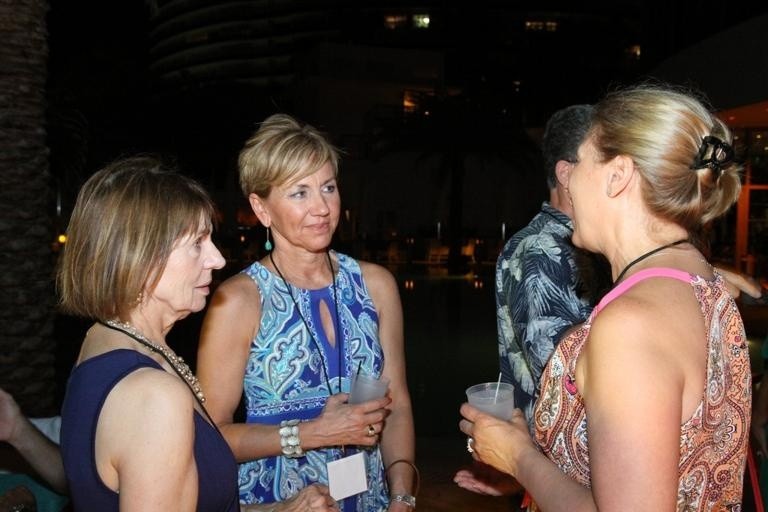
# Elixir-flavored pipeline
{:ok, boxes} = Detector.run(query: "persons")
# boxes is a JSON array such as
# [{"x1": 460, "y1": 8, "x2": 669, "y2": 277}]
[
  {"x1": 494, "y1": 106, "x2": 761, "y2": 421},
  {"x1": 50, "y1": 154, "x2": 341, "y2": 512},
  {"x1": 194, "y1": 113, "x2": 418, "y2": 512},
  {"x1": 0, "y1": 389, "x2": 71, "y2": 512},
  {"x1": 454, "y1": 84, "x2": 753, "y2": 511}
]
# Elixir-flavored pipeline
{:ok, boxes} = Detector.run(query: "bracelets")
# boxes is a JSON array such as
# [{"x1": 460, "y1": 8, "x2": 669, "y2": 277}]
[{"x1": 279, "y1": 418, "x2": 305, "y2": 458}]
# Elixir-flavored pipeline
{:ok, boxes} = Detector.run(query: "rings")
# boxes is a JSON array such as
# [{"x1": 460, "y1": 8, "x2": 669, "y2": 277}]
[
  {"x1": 368, "y1": 424, "x2": 375, "y2": 437},
  {"x1": 467, "y1": 438, "x2": 473, "y2": 452},
  {"x1": 12, "y1": 504, "x2": 25, "y2": 512}
]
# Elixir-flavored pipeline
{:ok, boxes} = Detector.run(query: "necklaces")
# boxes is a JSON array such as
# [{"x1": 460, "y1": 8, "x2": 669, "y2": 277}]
[
  {"x1": 614, "y1": 239, "x2": 691, "y2": 285},
  {"x1": 105, "y1": 315, "x2": 206, "y2": 403}
]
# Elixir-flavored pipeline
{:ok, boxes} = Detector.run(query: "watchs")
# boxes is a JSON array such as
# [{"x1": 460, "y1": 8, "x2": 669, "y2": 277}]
[{"x1": 390, "y1": 494, "x2": 417, "y2": 506}]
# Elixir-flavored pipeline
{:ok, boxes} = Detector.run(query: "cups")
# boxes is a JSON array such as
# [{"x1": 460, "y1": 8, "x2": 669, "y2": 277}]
[
  {"x1": 466, "y1": 382, "x2": 514, "y2": 479},
  {"x1": 347, "y1": 371, "x2": 389, "y2": 403}
]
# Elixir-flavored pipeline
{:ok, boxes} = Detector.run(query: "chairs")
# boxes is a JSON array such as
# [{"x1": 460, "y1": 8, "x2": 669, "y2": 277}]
[{"x1": 339, "y1": 232, "x2": 449, "y2": 266}]
